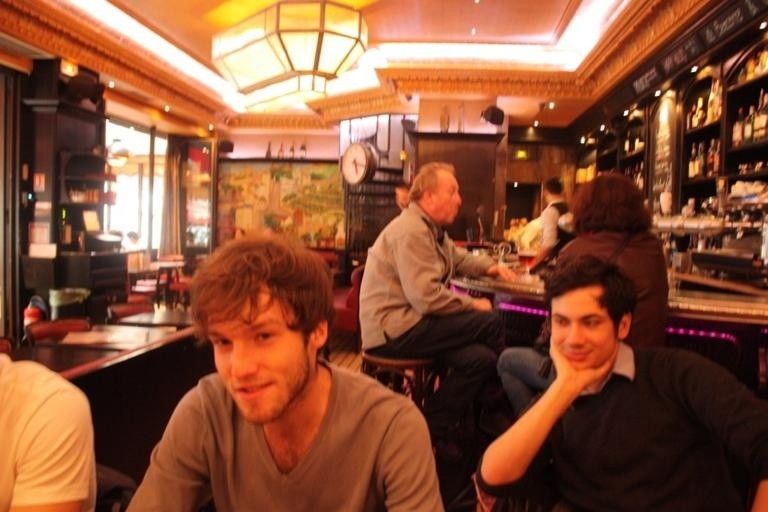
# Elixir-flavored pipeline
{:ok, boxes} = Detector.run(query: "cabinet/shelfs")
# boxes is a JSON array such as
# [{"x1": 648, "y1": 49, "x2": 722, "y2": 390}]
[
  {"x1": 21, "y1": 98, "x2": 110, "y2": 250},
  {"x1": 575, "y1": 11, "x2": 768, "y2": 287}
]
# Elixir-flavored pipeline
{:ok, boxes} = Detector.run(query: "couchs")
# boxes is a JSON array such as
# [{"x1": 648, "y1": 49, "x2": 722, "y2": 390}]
[{"x1": 332, "y1": 265, "x2": 365, "y2": 354}]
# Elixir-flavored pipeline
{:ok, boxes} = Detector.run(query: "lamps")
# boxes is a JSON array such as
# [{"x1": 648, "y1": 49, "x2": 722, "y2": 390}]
[{"x1": 209, "y1": 0, "x2": 368, "y2": 113}]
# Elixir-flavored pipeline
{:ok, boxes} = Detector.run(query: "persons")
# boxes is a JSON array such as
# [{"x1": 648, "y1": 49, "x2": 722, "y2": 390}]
[
  {"x1": 475, "y1": 250, "x2": 768, "y2": 512},
  {"x1": 377, "y1": 181, "x2": 414, "y2": 235},
  {"x1": 0, "y1": 350, "x2": 96, "y2": 512},
  {"x1": 495, "y1": 170, "x2": 669, "y2": 417},
  {"x1": 517, "y1": 177, "x2": 575, "y2": 273},
  {"x1": 123, "y1": 226, "x2": 444, "y2": 512},
  {"x1": 356, "y1": 159, "x2": 517, "y2": 458}
]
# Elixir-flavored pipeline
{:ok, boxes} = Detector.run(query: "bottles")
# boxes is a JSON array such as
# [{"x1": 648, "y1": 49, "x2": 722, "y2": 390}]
[
  {"x1": 624, "y1": 129, "x2": 640, "y2": 154},
  {"x1": 707, "y1": 79, "x2": 722, "y2": 124},
  {"x1": 456, "y1": 100, "x2": 465, "y2": 134},
  {"x1": 686, "y1": 97, "x2": 706, "y2": 130},
  {"x1": 439, "y1": 103, "x2": 448, "y2": 135},
  {"x1": 264, "y1": 140, "x2": 310, "y2": 159},
  {"x1": 731, "y1": 87, "x2": 768, "y2": 147},
  {"x1": 691, "y1": 232, "x2": 712, "y2": 277},
  {"x1": 58, "y1": 207, "x2": 71, "y2": 249},
  {"x1": 623, "y1": 161, "x2": 643, "y2": 186},
  {"x1": 77, "y1": 230, "x2": 86, "y2": 251},
  {"x1": 687, "y1": 139, "x2": 721, "y2": 177}
]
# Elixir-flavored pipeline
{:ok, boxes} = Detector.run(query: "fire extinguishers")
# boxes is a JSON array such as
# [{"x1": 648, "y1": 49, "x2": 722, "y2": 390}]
[{"x1": 23, "y1": 295, "x2": 49, "y2": 335}]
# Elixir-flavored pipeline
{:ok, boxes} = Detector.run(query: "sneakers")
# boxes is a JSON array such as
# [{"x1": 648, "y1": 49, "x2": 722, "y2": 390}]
[{"x1": 428, "y1": 420, "x2": 480, "y2": 441}]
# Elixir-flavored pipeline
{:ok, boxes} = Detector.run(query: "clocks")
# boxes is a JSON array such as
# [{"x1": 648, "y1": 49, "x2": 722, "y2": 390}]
[{"x1": 341, "y1": 143, "x2": 377, "y2": 187}]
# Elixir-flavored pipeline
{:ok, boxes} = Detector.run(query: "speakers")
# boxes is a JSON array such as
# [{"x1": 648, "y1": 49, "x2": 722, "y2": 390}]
[
  {"x1": 481, "y1": 105, "x2": 504, "y2": 124},
  {"x1": 220, "y1": 140, "x2": 234, "y2": 152}
]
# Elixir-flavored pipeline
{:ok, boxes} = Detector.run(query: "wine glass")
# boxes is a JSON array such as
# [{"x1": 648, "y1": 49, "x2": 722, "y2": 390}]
[{"x1": 518, "y1": 239, "x2": 537, "y2": 282}]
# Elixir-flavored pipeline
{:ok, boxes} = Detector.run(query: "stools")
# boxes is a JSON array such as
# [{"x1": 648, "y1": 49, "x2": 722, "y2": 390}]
[
  {"x1": 0, "y1": 254, "x2": 193, "y2": 353},
  {"x1": 358, "y1": 351, "x2": 440, "y2": 410}
]
